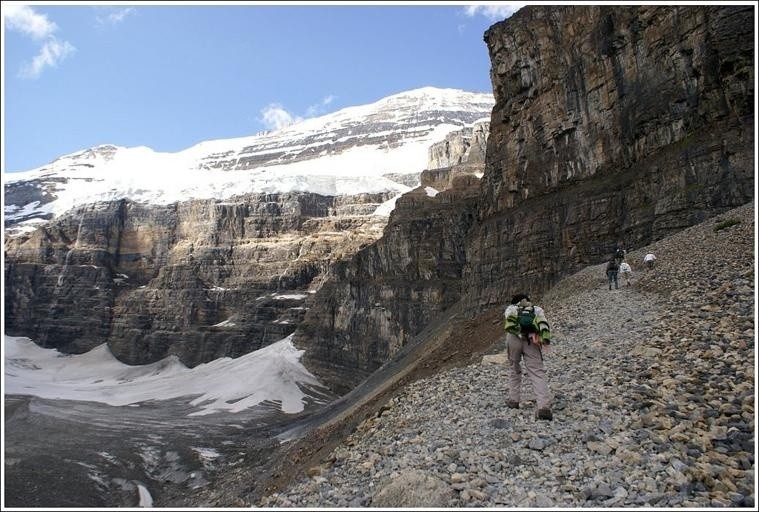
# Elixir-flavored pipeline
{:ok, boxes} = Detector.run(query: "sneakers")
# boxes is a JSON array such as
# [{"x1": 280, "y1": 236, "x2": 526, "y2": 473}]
[
  {"x1": 534, "y1": 407, "x2": 552, "y2": 421},
  {"x1": 505, "y1": 398, "x2": 519, "y2": 409}
]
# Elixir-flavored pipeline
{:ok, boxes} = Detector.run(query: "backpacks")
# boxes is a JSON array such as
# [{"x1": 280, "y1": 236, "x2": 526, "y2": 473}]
[
  {"x1": 516, "y1": 304, "x2": 539, "y2": 335},
  {"x1": 607, "y1": 250, "x2": 629, "y2": 272}
]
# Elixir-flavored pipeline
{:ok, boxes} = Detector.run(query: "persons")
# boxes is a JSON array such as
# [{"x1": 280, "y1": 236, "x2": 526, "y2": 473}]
[
  {"x1": 606, "y1": 248, "x2": 657, "y2": 290},
  {"x1": 505, "y1": 294, "x2": 552, "y2": 420}
]
